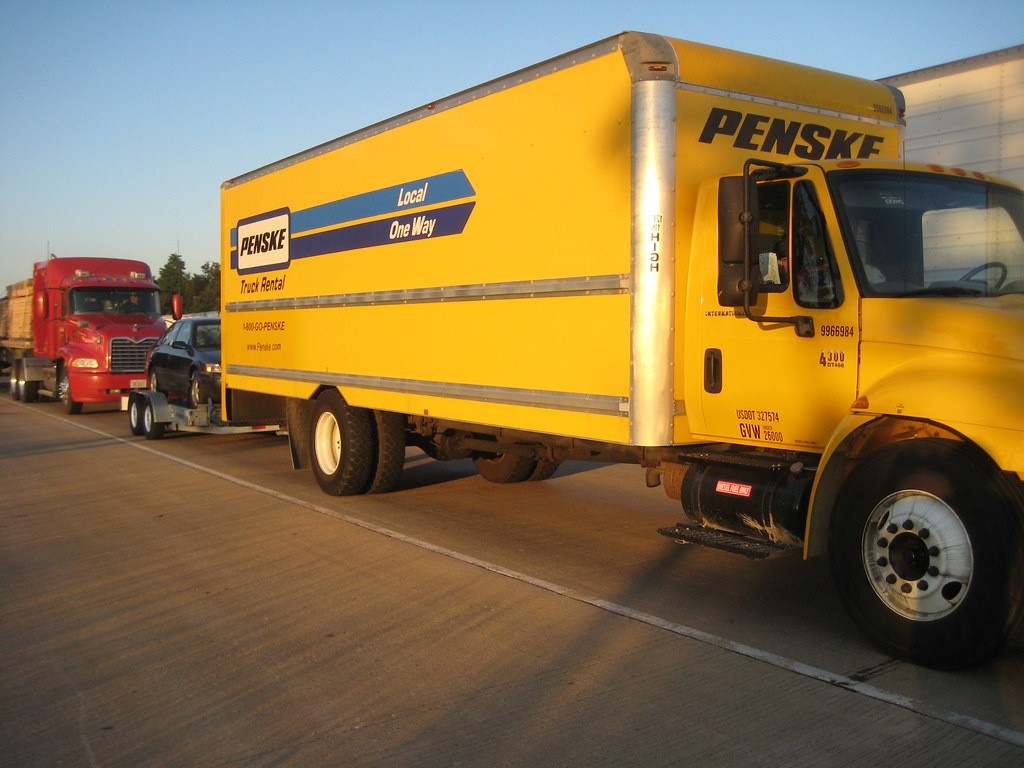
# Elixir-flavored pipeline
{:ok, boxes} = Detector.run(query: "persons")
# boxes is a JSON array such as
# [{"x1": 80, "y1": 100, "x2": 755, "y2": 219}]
[
  {"x1": 754, "y1": 232, "x2": 827, "y2": 303},
  {"x1": 119, "y1": 291, "x2": 146, "y2": 313}
]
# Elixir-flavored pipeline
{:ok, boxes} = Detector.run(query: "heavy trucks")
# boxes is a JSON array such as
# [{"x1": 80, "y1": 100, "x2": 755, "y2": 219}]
[{"x1": 0, "y1": 256, "x2": 182, "y2": 416}]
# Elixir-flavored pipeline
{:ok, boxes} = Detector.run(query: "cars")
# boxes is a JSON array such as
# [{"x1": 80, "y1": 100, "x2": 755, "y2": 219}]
[{"x1": 143, "y1": 315, "x2": 226, "y2": 405}]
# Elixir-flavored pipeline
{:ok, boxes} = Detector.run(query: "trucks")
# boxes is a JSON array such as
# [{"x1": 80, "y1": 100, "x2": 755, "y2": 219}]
[{"x1": 214, "y1": 29, "x2": 1023, "y2": 674}]
